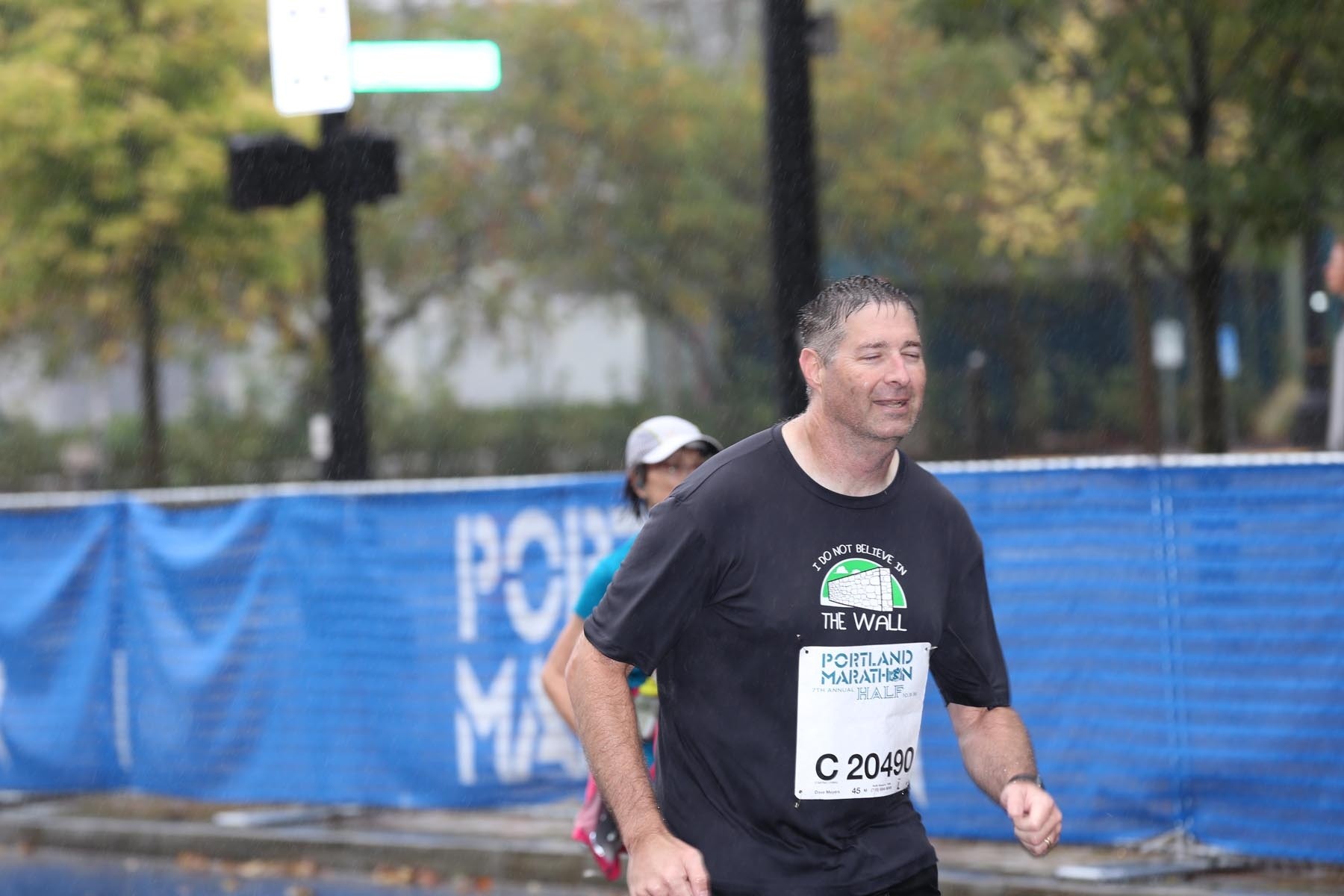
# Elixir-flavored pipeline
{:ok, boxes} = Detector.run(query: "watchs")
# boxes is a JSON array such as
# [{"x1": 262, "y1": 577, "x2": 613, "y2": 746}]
[{"x1": 1008, "y1": 772, "x2": 1045, "y2": 790}]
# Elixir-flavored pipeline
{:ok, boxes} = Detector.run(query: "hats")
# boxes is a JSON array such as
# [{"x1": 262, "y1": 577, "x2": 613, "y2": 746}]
[{"x1": 625, "y1": 415, "x2": 724, "y2": 464}]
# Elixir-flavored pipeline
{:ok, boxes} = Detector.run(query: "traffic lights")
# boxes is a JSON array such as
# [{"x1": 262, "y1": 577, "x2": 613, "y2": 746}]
[{"x1": 227, "y1": 130, "x2": 403, "y2": 209}]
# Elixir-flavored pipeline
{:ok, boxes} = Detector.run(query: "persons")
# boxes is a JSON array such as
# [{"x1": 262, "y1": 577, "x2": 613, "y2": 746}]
[
  {"x1": 542, "y1": 416, "x2": 723, "y2": 879},
  {"x1": 568, "y1": 276, "x2": 1061, "y2": 896}
]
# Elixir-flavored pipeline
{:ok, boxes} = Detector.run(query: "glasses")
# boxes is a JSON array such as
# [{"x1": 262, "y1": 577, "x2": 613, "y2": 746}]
[{"x1": 662, "y1": 461, "x2": 700, "y2": 475}]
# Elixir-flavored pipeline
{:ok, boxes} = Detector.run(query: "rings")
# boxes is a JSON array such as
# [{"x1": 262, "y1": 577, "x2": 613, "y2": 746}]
[{"x1": 1044, "y1": 838, "x2": 1051, "y2": 848}]
